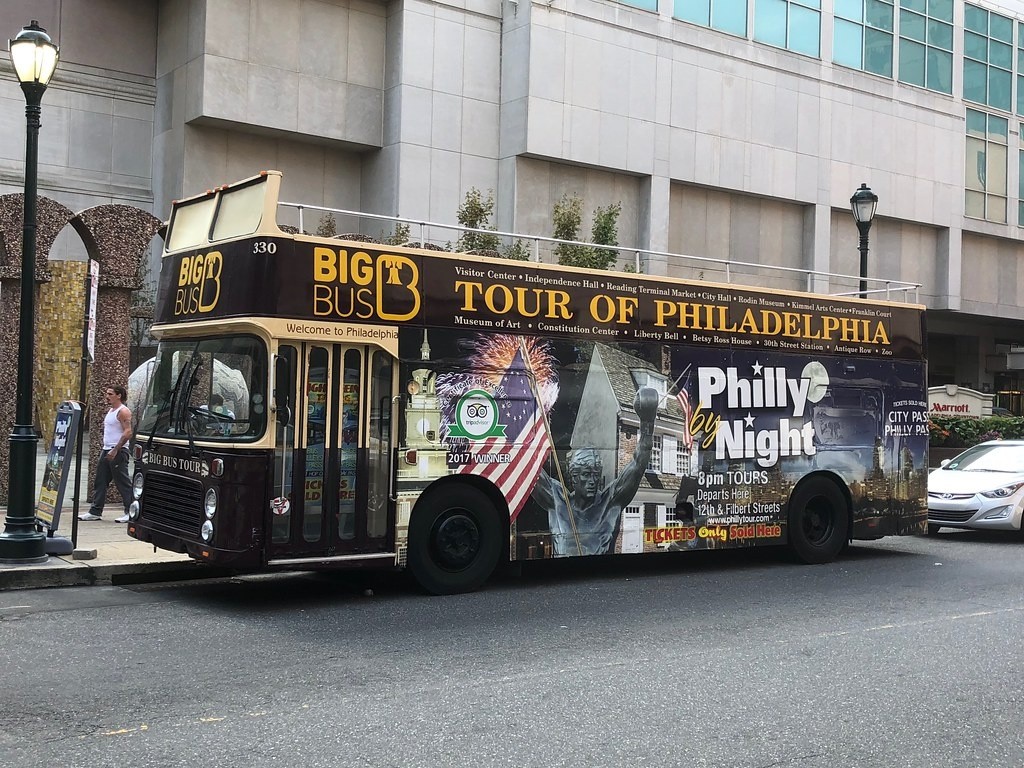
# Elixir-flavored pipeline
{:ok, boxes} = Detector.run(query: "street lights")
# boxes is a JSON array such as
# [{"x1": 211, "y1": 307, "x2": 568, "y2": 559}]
[
  {"x1": 0, "y1": 19, "x2": 62, "y2": 565},
  {"x1": 848, "y1": 180, "x2": 878, "y2": 301}
]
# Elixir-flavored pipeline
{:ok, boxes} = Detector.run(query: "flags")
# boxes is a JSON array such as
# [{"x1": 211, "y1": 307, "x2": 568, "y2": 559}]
[
  {"x1": 676, "y1": 369, "x2": 698, "y2": 450},
  {"x1": 455, "y1": 348, "x2": 550, "y2": 525}
]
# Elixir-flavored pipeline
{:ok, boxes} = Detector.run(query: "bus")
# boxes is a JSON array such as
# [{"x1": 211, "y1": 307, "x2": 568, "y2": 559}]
[{"x1": 127, "y1": 171, "x2": 929, "y2": 599}]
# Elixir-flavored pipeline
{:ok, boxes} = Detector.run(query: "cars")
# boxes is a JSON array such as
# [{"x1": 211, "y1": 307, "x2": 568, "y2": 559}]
[{"x1": 924, "y1": 438, "x2": 1024, "y2": 537}]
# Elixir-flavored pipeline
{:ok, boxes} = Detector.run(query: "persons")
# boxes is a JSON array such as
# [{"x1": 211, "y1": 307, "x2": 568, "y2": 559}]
[
  {"x1": 78, "y1": 386, "x2": 135, "y2": 523},
  {"x1": 190, "y1": 393, "x2": 236, "y2": 430},
  {"x1": 531, "y1": 387, "x2": 659, "y2": 558}
]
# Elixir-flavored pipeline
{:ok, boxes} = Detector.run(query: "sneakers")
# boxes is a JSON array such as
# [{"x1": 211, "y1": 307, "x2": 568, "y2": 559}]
[
  {"x1": 115, "y1": 514, "x2": 129, "y2": 523},
  {"x1": 78, "y1": 512, "x2": 102, "y2": 521}
]
